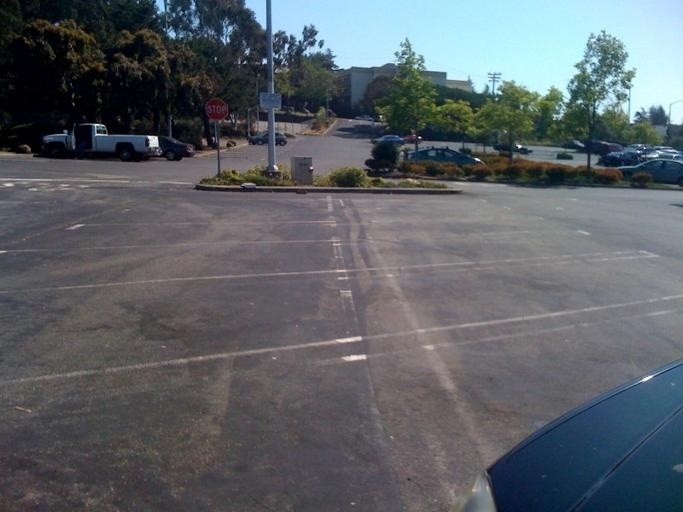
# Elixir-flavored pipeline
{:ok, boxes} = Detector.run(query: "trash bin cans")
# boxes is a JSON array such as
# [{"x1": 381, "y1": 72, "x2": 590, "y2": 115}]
[{"x1": 291, "y1": 156, "x2": 313, "y2": 184}]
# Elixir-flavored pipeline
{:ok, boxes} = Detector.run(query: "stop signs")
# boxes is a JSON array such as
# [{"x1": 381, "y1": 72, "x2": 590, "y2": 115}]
[{"x1": 206, "y1": 98, "x2": 229, "y2": 122}]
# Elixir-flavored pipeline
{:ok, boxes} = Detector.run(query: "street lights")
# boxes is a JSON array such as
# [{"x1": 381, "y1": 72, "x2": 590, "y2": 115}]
[{"x1": 486, "y1": 71, "x2": 502, "y2": 96}]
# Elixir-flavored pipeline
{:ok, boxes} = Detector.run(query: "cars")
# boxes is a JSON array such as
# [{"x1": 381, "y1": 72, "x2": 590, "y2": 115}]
[
  {"x1": 401, "y1": 146, "x2": 488, "y2": 180},
  {"x1": 156, "y1": 135, "x2": 196, "y2": 162},
  {"x1": 247, "y1": 131, "x2": 289, "y2": 146},
  {"x1": 400, "y1": 134, "x2": 423, "y2": 144},
  {"x1": 368, "y1": 133, "x2": 406, "y2": 146},
  {"x1": 493, "y1": 142, "x2": 533, "y2": 155},
  {"x1": 593, "y1": 138, "x2": 683, "y2": 167},
  {"x1": 460, "y1": 357, "x2": 683, "y2": 511},
  {"x1": 354, "y1": 113, "x2": 375, "y2": 121},
  {"x1": 616, "y1": 157, "x2": 683, "y2": 187},
  {"x1": 561, "y1": 139, "x2": 585, "y2": 148}
]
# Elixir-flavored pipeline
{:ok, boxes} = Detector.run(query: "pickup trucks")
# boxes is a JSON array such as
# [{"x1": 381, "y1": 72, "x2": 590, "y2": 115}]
[{"x1": 40, "y1": 121, "x2": 162, "y2": 162}]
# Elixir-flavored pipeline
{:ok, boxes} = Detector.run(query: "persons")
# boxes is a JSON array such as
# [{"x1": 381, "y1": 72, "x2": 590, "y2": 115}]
[{"x1": 107, "y1": 106, "x2": 167, "y2": 134}]
